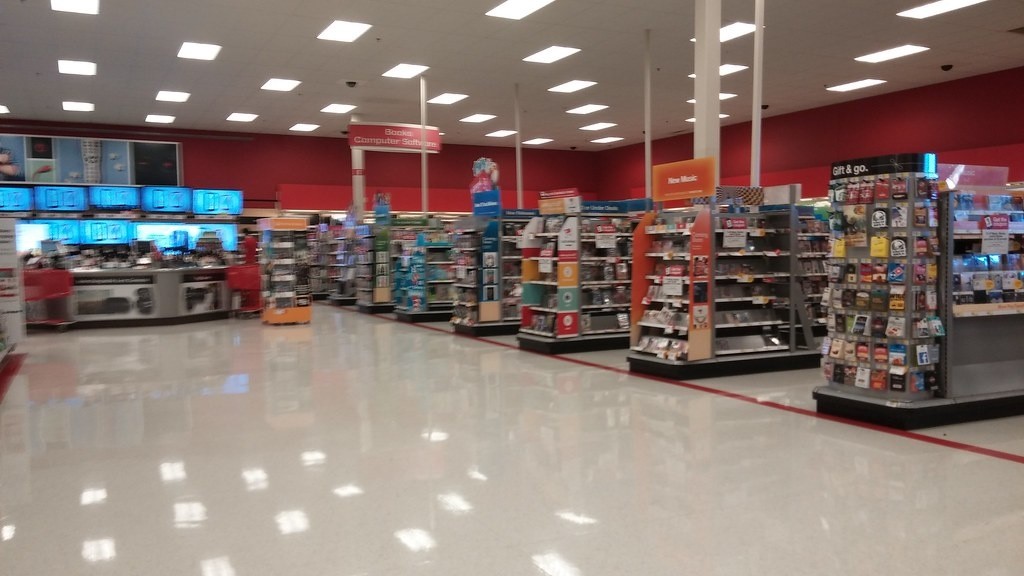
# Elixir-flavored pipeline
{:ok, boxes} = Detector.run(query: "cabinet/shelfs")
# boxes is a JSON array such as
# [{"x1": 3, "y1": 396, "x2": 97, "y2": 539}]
[
  {"x1": 257, "y1": 218, "x2": 314, "y2": 325},
  {"x1": 308, "y1": 219, "x2": 537, "y2": 338},
  {"x1": 813, "y1": 153, "x2": 1024, "y2": 432},
  {"x1": 515, "y1": 184, "x2": 828, "y2": 381}
]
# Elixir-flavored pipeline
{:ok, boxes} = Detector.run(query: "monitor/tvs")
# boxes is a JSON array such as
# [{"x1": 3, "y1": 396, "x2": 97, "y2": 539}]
[{"x1": 0, "y1": 182, "x2": 244, "y2": 258}]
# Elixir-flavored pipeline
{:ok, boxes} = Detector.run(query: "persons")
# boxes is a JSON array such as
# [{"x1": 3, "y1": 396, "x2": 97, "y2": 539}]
[{"x1": 240, "y1": 228, "x2": 258, "y2": 264}]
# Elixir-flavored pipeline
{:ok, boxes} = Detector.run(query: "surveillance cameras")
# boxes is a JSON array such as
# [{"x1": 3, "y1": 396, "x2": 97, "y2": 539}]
[
  {"x1": 346, "y1": 82, "x2": 356, "y2": 87},
  {"x1": 941, "y1": 65, "x2": 953, "y2": 71},
  {"x1": 570, "y1": 146, "x2": 576, "y2": 150},
  {"x1": 342, "y1": 131, "x2": 348, "y2": 135},
  {"x1": 761, "y1": 105, "x2": 769, "y2": 109}
]
